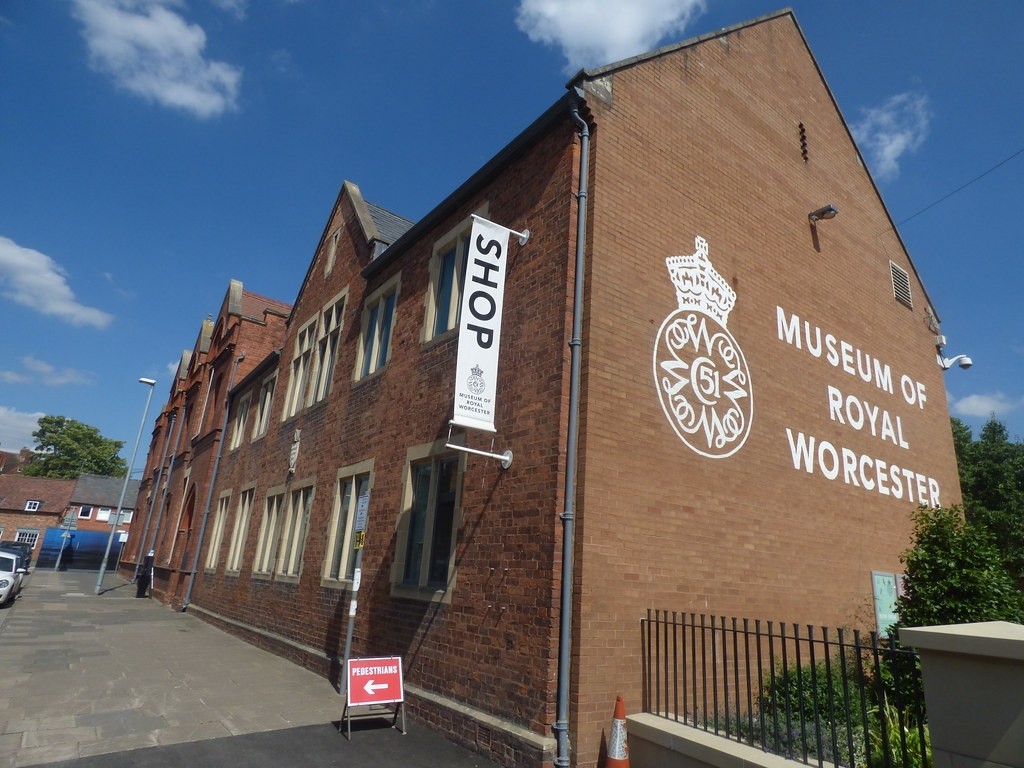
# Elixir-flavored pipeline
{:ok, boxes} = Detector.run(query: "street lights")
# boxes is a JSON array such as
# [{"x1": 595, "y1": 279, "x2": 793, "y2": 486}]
[{"x1": 93, "y1": 377, "x2": 158, "y2": 597}]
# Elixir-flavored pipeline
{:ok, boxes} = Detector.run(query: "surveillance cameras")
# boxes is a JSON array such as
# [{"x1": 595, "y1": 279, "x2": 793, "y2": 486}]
[{"x1": 959, "y1": 357, "x2": 973, "y2": 368}]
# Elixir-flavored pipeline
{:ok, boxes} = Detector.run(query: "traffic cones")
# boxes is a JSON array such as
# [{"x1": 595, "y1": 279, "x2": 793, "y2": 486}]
[{"x1": 603, "y1": 694, "x2": 632, "y2": 768}]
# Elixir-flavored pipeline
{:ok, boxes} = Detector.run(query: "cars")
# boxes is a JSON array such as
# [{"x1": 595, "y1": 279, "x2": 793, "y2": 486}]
[
  {"x1": 0, "y1": 552, "x2": 27, "y2": 606},
  {"x1": 0, "y1": 541, "x2": 32, "y2": 575}
]
